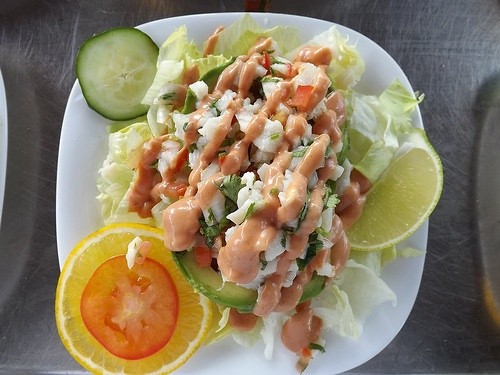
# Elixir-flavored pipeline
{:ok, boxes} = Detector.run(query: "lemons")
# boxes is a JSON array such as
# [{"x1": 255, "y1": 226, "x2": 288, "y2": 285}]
[
  {"x1": 339, "y1": 128, "x2": 443, "y2": 251},
  {"x1": 76, "y1": 27, "x2": 161, "y2": 122}
]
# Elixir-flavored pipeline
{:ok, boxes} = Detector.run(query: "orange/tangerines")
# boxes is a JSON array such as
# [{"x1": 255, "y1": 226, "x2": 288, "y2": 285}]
[{"x1": 57, "y1": 222, "x2": 213, "y2": 375}]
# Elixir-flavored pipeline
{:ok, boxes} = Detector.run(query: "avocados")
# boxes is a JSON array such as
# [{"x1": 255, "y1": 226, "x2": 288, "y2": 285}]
[{"x1": 172, "y1": 240, "x2": 325, "y2": 307}]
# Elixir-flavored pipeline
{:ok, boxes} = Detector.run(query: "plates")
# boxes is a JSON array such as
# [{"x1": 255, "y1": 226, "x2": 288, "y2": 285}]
[{"x1": 55, "y1": 13, "x2": 435, "y2": 375}]
